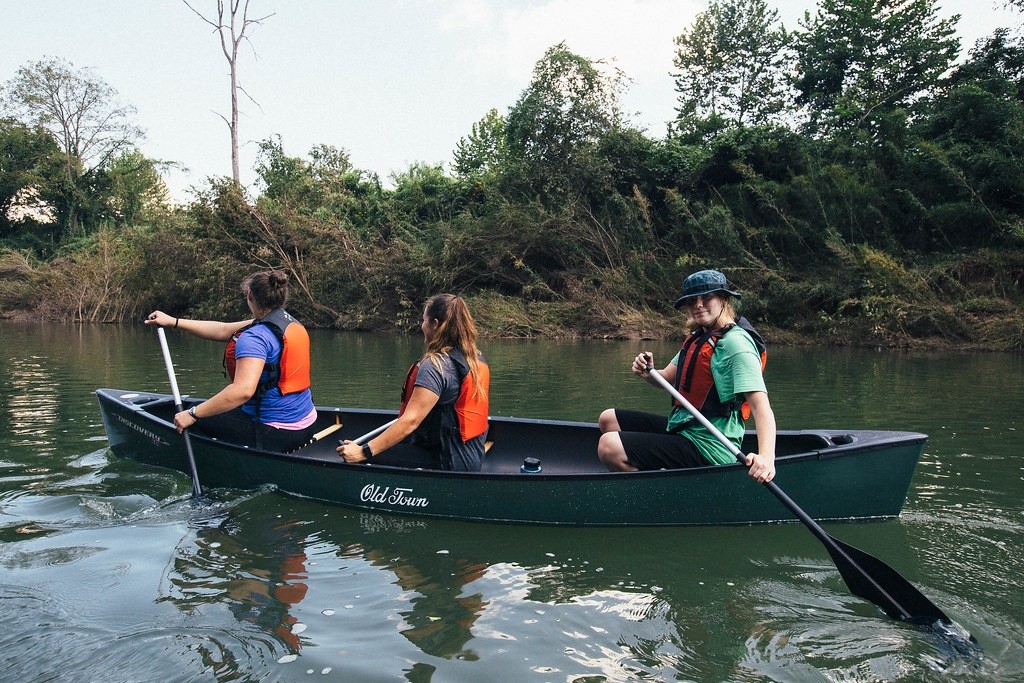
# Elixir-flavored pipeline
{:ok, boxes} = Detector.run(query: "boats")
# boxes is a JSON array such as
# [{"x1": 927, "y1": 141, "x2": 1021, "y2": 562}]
[{"x1": 95, "y1": 385, "x2": 928, "y2": 529}]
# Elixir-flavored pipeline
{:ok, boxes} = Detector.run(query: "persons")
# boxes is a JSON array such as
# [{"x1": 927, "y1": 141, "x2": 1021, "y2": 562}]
[
  {"x1": 144, "y1": 269, "x2": 320, "y2": 452},
  {"x1": 336, "y1": 292, "x2": 491, "y2": 471},
  {"x1": 597, "y1": 269, "x2": 778, "y2": 484}
]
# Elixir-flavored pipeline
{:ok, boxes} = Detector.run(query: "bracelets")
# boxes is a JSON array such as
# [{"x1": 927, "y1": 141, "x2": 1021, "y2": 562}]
[{"x1": 174, "y1": 318, "x2": 178, "y2": 329}]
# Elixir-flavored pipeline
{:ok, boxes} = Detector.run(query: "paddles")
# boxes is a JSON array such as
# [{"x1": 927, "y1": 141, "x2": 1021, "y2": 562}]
[
  {"x1": 149, "y1": 316, "x2": 218, "y2": 508},
  {"x1": 641, "y1": 347, "x2": 980, "y2": 648},
  {"x1": 338, "y1": 411, "x2": 399, "y2": 450}
]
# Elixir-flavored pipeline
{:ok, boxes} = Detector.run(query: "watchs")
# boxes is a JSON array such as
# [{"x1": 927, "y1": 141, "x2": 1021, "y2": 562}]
[
  {"x1": 188, "y1": 406, "x2": 200, "y2": 421},
  {"x1": 362, "y1": 444, "x2": 372, "y2": 460}
]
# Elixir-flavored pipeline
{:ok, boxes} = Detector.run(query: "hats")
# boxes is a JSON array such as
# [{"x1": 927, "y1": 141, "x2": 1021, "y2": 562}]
[{"x1": 674, "y1": 270, "x2": 742, "y2": 311}]
